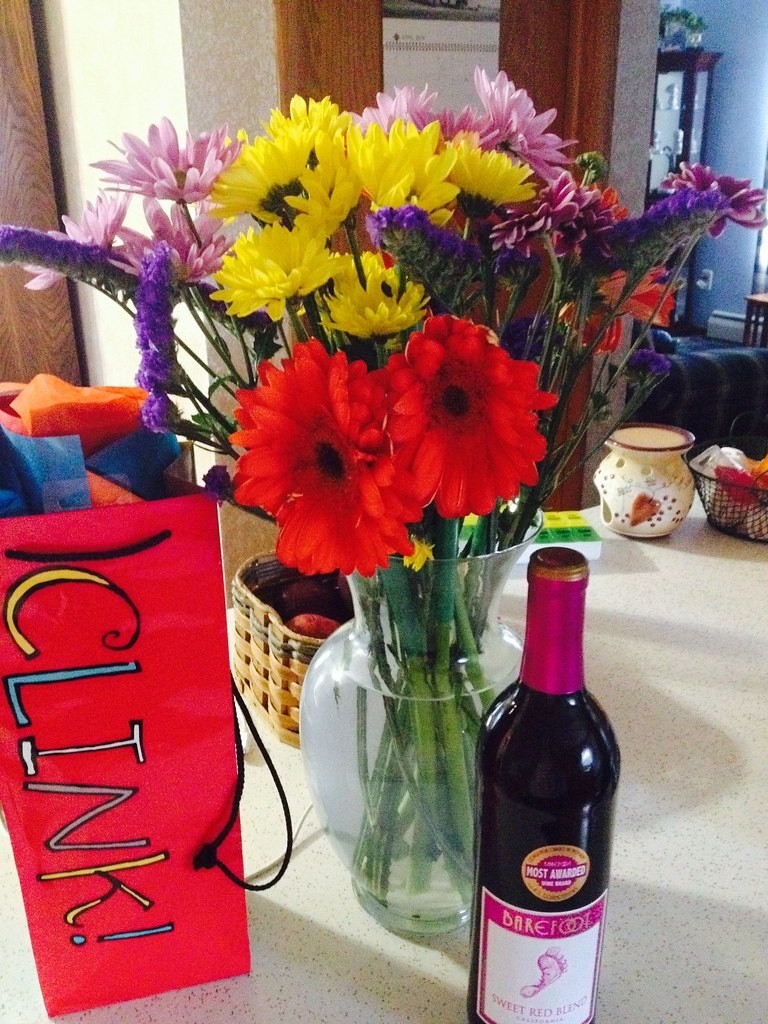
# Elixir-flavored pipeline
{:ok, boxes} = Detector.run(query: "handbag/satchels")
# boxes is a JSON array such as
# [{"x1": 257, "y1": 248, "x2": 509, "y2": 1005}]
[{"x1": 0, "y1": 475, "x2": 294, "y2": 1019}]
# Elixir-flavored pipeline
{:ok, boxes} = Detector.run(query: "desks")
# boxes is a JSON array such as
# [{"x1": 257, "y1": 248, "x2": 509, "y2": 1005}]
[{"x1": 1, "y1": 481, "x2": 768, "y2": 1024}]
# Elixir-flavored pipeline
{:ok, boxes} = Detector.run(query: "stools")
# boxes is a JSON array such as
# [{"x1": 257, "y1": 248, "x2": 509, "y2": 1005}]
[{"x1": 742, "y1": 292, "x2": 768, "y2": 348}]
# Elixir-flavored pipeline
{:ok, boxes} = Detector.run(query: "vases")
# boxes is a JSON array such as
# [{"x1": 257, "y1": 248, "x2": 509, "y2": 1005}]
[{"x1": 299, "y1": 499, "x2": 547, "y2": 937}]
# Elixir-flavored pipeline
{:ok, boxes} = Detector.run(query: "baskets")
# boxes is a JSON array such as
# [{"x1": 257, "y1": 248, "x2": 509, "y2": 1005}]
[
  {"x1": 232, "y1": 550, "x2": 328, "y2": 750},
  {"x1": 684, "y1": 411, "x2": 768, "y2": 545}
]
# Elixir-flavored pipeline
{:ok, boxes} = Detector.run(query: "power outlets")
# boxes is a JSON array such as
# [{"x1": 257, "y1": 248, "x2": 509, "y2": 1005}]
[{"x1": 701, "y1": 269, "x2": 713, "y2": 291}]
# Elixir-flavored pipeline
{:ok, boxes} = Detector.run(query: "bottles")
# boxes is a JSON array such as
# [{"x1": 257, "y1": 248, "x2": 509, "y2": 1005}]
[
  {"x1": 595, "y1": 423, "x2": 698, "y2": 541},
  {"x1": 465, "y1": 548, "x2": 619, "y2": 1024}
]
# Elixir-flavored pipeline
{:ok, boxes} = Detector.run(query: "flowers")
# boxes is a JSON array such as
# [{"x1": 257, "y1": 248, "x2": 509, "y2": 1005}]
[{"x1": 1, "y1": 65, "x2": 768, "y2": 918}]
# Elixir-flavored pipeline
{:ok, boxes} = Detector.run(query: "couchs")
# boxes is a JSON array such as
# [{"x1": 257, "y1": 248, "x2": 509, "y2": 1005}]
[{"x1": 626, "y1": 317, "x2": 768, "y2": 436}]
